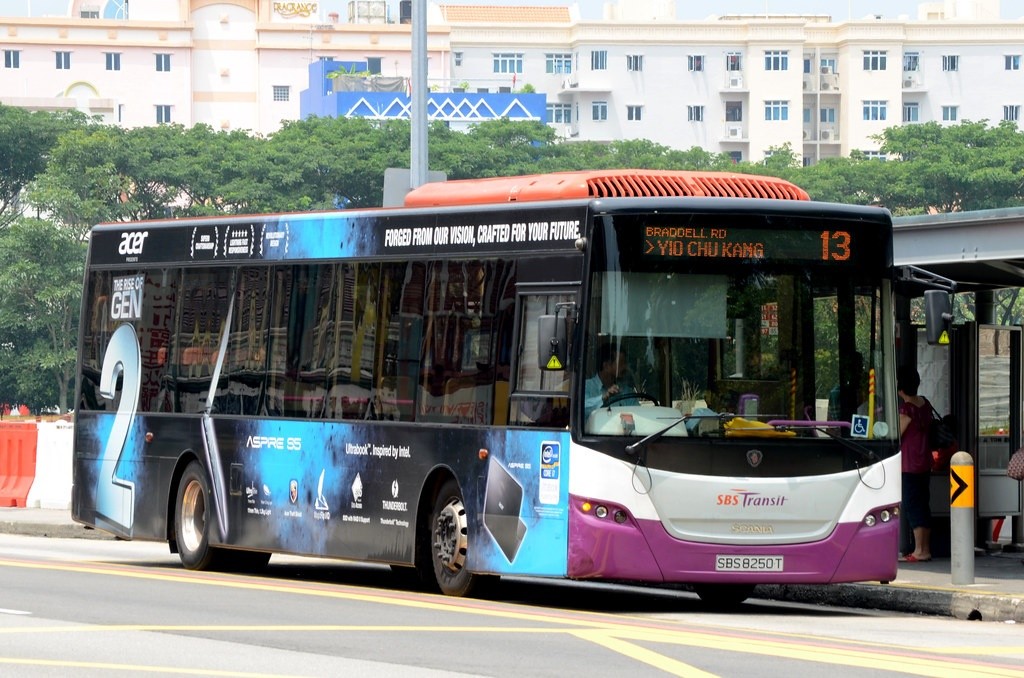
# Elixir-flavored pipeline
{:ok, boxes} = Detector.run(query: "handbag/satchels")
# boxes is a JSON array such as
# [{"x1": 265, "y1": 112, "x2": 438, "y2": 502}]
[
  {"x1": 1008, "y1": 448, "x2": 1024, "y2": 480},
  {"x1": 920, "y1": 396, "x2": 954, "y2": 449}
]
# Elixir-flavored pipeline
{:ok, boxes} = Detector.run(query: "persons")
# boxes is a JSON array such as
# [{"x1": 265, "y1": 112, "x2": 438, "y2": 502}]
[
  {"x1": 585, "y1": 344, "x2": 640, "y2": 416},
  {"x1": 826, "y1": 349, "x2": 877, "y2": 436},
  {"x1": 897, "y1": 366, "x2": 939, "y2": 562}
]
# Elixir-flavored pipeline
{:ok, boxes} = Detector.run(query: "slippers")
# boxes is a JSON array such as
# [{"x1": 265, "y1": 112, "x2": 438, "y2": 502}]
[{"x1": 898, "y1": 554, "x2": 932, "y2": 562}]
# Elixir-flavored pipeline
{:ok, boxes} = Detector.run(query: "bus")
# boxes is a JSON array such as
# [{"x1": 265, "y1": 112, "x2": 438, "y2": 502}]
[{"x1": 70, "y1": 169, "x2": 958, "y2": 602}]
